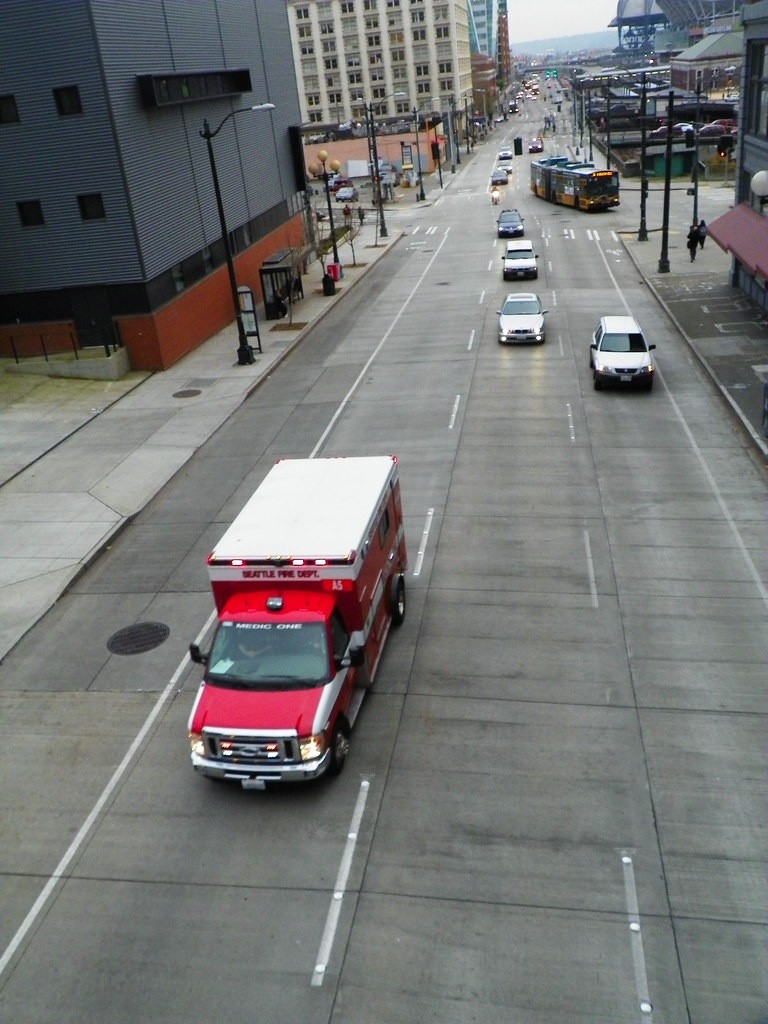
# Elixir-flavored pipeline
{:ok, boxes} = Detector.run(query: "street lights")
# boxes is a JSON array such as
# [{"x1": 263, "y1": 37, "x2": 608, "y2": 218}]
[
  {"x1": 307, "y1": 148, "x2": 340, "y2": 265},
  {"x1": 358, "y1": 92, "x2": 404, "y2": 238},
  {"x1": 197, "y1": 102, "x2": 277, "y2": 365},
  {"x1": 411, "y1": 97, "x2": 439, "y2": 200}
]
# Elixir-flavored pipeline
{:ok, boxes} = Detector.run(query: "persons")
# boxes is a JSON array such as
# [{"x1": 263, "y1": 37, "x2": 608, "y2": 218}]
[
  {"x1": 343, "y1": 204, "x2": 350, "y2": 226},
  {"x1": 358, "y1": 207, "x2": 364, "y2": 225},
  {"x1": 698, "y1": 220, "x2": 707, "y2": 249},
  {"x1": 687, "y1": 224, "x2": 698, "y2": 262},
  {"x1": 276, "y1": 290, "x2": 289, "y2": 318},
  {"x1": 491, "y1": 186, "x2": 499, "y2": 196}
]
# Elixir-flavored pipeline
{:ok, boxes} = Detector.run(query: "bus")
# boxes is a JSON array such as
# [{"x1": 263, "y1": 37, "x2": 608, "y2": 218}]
[{"x1": 530, "y1": 156, "x2": 620, "y2": 211}]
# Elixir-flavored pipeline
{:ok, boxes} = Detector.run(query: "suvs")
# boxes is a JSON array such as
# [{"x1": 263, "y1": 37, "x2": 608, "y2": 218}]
[
  {"x1": 324, "y1": 179, "x2": 353, "y2": 191},
  {"x1": 590, "y1": 316, "x2": 656, "y2": 392}
]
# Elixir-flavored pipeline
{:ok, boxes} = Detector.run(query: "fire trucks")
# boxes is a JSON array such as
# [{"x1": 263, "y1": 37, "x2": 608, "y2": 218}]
[{"x1": 186, "y1": 457, "x2": 408, "y2": 792}]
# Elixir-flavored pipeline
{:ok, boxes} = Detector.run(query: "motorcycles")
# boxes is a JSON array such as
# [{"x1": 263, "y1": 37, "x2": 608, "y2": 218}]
[{"x1": 492, "y1": 191, "x2": 499, "y2": 205}]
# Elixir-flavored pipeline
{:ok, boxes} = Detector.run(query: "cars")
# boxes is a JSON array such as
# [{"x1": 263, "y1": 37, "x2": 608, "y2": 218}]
[
  {"x1": 335, "y1": 188, "x2": 359, "y2": 203},
  {"x1": 497, "y1": 209, "x2": 524, "y2": 237},
  {"x1": 509, "y1": 72, "x2": 557, "y2": 114},
  {"x1": 491, "y1": 170, "x2": 509, "y2": 185},
  {"x1": 501, "y1": 240, "x2": 539, "y2": 279},
  {"x1": 499, "y1": 147, "x2": 512, "y2": 159},
  {"x1": 585, "y1": 98, "x2": 635, "y2": 117},
  {"x1": 650, "y1": 117, "x2": 738, "y2": 137},
  {"x1": 529, "y1": 137, "x2": 542, "y2": 153},
  {"x1": 496, "y1": 293, "x2": 548, "y2": 344},
  {"x1": 498, "y1": 160, "x2": 512, "y2": 173}
]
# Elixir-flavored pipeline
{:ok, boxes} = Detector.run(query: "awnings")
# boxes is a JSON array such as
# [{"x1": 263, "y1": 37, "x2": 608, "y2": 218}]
[{"x1": 707, "y1": 201, "x2": 768, "y2": 281}]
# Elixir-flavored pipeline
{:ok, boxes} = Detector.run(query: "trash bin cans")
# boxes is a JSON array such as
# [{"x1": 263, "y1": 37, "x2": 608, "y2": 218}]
[{"x1": 327, "y1": 264, "x2": 341, "y2": 282}]
